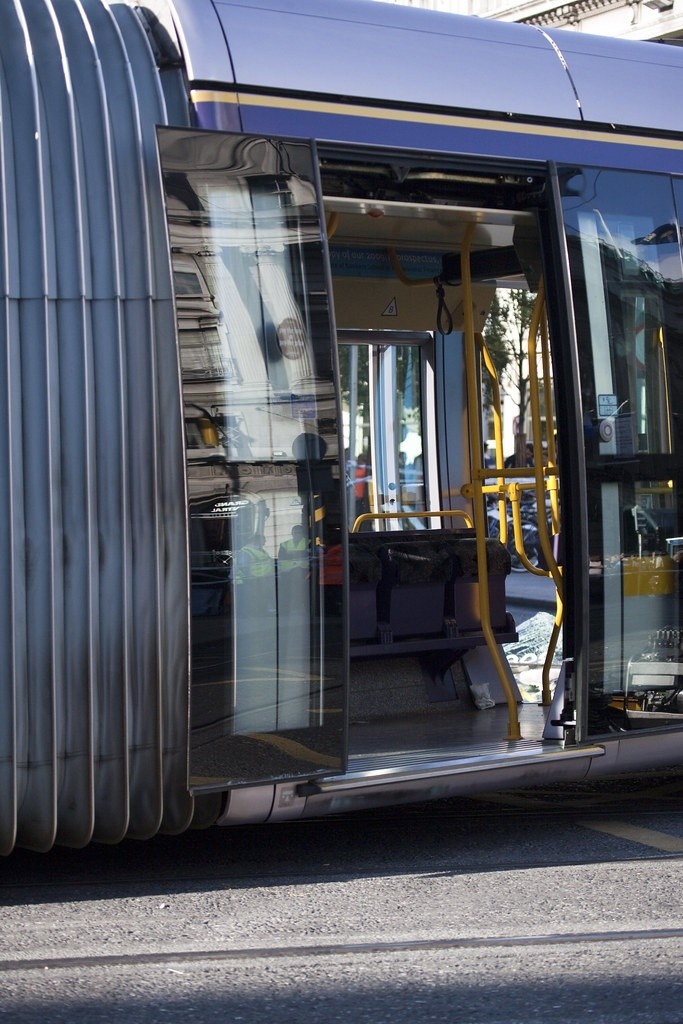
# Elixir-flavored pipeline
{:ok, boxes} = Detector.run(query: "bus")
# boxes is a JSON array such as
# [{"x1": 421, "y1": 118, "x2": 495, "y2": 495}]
[{"x1": 0, "y1": 2, "x2": 683, "y2": 858}]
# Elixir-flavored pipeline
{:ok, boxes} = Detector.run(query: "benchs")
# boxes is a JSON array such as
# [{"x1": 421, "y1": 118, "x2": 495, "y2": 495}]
[{"x1": 350, "y1": 529, "x2": 518, "y2": 657}]
